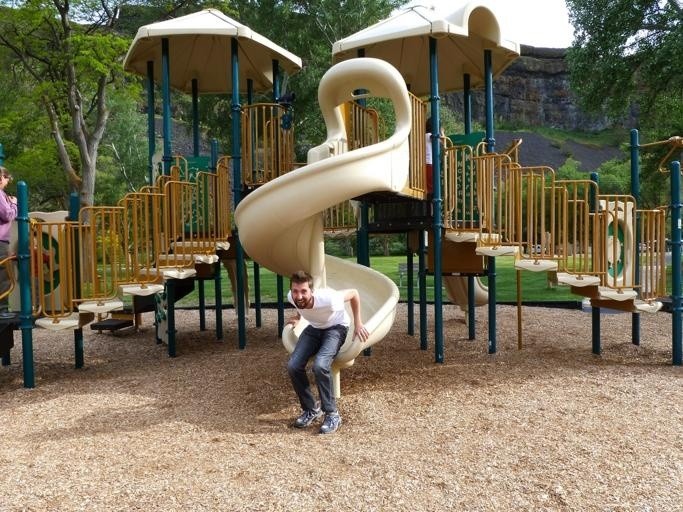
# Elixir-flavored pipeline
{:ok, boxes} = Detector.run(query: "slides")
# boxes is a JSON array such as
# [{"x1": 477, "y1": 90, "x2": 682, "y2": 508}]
[
  {"x1": 441, "y1": 275, "x2": 488, "y2": 307},
  {"x1": 234, "y1": 57, "x2": 409, "y2": 367}
]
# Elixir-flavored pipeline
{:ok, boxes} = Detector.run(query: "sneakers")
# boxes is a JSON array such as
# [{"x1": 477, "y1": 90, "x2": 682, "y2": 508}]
[
  {"x1": 321, "y1": 413, "x2": 342, "y2": 434},
  {"x1": 0, "y1": 312, "x2": 16, "y2": 319},
  {"x1": 295, "y1": 408, "x2": 322, "y2": 427}
]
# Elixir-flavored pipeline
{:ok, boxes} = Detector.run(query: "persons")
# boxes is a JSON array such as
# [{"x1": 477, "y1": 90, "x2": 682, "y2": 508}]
[
  {"x1": 0, "y1": 165, "x2": 18, "y2": 314},
  {"x1": 10, "y1": 235, "x2": 55, "y2": 318},
  {"x1": 425, "y1": 115, "x2": 445, "y2": 194},
  {"x1": 283, "y1": 270, "x2": 370, "y2": 434}
]
